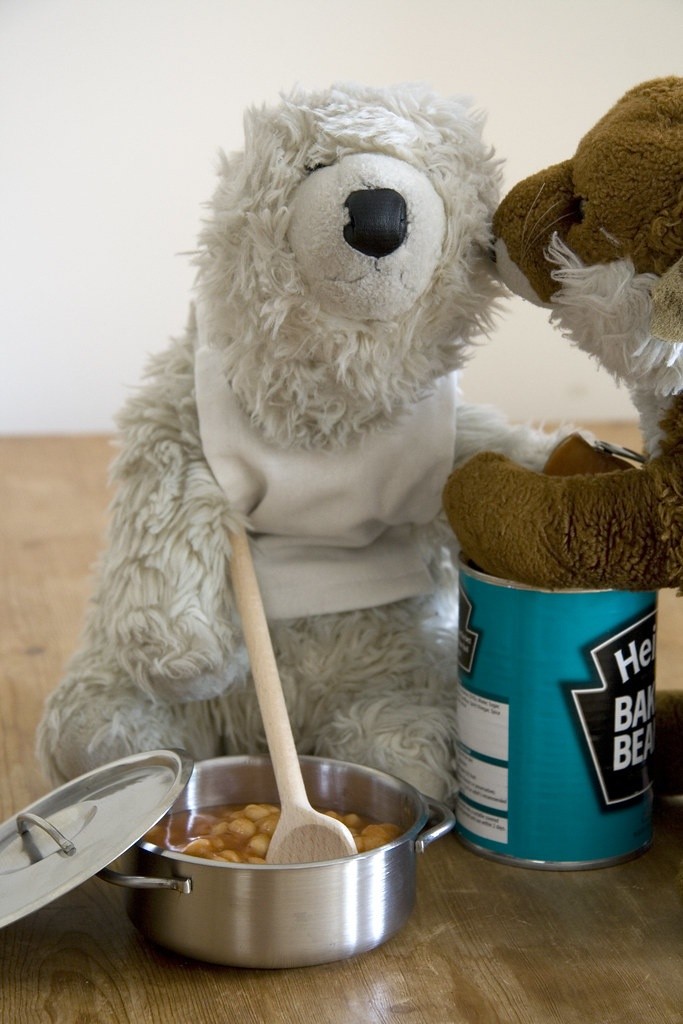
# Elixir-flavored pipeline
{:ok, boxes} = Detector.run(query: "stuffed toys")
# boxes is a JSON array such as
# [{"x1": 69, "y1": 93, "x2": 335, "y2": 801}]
[
  {"x1": 441, "y1": 75, "x2": 683, "y2": 798},
  {"x1": 36, "y1": 76, "x2": 599, "y2": 820}
]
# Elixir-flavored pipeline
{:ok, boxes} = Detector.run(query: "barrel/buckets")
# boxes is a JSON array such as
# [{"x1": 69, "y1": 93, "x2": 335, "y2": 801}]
[{"x1": 454, "y1": 549, "x2": 660, "y2": 872}]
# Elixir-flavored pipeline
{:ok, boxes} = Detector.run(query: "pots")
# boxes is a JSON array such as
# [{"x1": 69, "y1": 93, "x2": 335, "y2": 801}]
[{"x1": 93, "y1": 752, "x2": 459, "y2": 971}]
